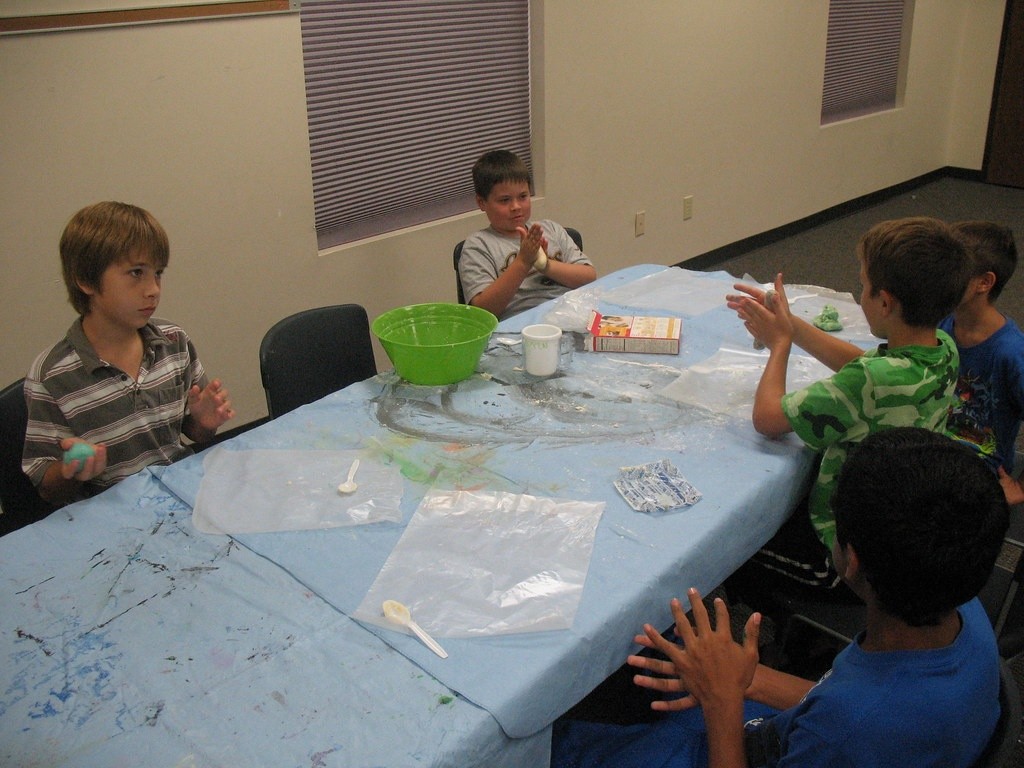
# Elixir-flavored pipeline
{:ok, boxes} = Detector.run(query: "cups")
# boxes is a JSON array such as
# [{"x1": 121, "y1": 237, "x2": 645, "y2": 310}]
[{"x1": 521, "y1": 324, "x2": 563, "y2": 377}]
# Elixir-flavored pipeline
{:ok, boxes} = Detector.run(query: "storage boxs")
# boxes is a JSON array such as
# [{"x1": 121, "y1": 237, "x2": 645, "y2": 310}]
[{"x1": 586, "y1": 308, "x2": 682, "y2": 354}]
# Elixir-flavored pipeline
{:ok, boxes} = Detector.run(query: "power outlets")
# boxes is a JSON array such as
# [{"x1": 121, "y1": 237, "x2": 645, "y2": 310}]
[{"x1": 684, "y1": 195, "x2": 693, "y2": 220}]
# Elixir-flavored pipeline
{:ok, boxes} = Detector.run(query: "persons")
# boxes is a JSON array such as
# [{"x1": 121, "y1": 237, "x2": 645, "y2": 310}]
[
  {"x1": 436, "y1": 150, "x2": 597, "y2": 323},
  {"x1": 726, "y1": 215, "x2": 1024, "y2": 612},
  {"x1": 548, "y1": 426, "x2": 1000, "y2": 768},
  {"x1": 21, "y1": 201, "x2": 235, "y2": 506}
]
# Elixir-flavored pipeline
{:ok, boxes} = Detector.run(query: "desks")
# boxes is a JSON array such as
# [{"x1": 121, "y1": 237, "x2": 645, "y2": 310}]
[{"x1": 0, "y1": 262, "x2": 890, "y2": 768}]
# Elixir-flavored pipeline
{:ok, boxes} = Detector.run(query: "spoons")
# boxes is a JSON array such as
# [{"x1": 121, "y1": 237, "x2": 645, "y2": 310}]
[
  {"x1": 383, "y1": 600, "x2": 449, "y2": 659},
  {"x1": 338, "y1": 459, "x2": 360, "y2": 493}
]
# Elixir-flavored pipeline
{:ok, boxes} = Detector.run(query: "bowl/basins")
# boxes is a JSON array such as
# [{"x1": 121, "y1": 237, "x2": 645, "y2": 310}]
[{"x1": 371, "y1": 301, "x2": 499, "y2": 386}]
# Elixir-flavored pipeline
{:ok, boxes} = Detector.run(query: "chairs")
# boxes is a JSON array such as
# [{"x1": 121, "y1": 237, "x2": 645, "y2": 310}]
[
  {"x1": 778, "y1": 458, "x2": 1024, "y2": 768},
  {"x1": 0, "y1": 377, "x2": 39, "y2": 539},
  {"x1": 259, "y1": 304, "x2": 377, "y2": 420},
  {"x1": 454, "y1": 228, "x2": 583, "y2": 305}
]
